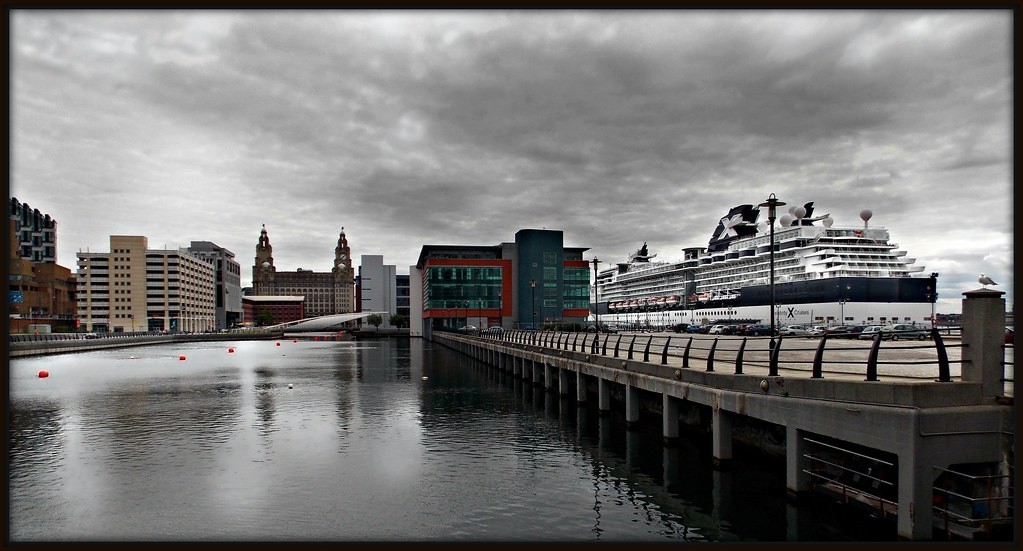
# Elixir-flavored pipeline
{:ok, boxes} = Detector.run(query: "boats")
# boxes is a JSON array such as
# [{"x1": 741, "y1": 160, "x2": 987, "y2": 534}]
[{"x1": 607, "y1": 292, "x2": 710, "y2": 312}]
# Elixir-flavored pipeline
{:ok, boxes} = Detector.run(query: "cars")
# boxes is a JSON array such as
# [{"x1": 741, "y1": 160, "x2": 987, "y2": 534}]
[
  {"x1": 671, "y1": 319, "x2": 929, "y2": 343},
  {"x1": 457, "y1": 325, "x2": 477, "y2": 333},
  {"x1": 1005, "y1": 327, "x2": 1015, "y2": 346},
  {"x1": 481, "y1": 326, "x2": 503, "y2": 334},
  {"x1": 583, "y1": 323, "x2": 619, "y2": 335}
]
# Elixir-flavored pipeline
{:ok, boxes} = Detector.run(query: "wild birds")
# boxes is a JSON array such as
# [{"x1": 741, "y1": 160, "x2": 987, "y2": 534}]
[{"x1": 977, "y1": 273, "x2": 999, "y2": 289}]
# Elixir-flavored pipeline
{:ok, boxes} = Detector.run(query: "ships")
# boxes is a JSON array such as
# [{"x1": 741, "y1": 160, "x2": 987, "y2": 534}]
[{"x1": 582, "y1": 198, "x2": 939, "y2": 337}]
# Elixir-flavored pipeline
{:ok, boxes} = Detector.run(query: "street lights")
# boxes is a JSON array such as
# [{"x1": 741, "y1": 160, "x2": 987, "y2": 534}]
[
  {"x1": 442, "y1": 307, "x2": 459, "y2": 330},
  {"x1": 497, "y1": 288, "x2": 504, "y2": 335},
  {"x1": 838, "y1": 298, "x2": 847, "y2": 326},
  {"x1": 588, "y1": 256, "x2": 603, "y2": 354},
  {"x1": 477, "y1": 297, "x2": 483, "y2": 337},
  {"x1": 464, "y1": 302, "x2": 468, "y2": 332},
  {"x1": 528, "y1": 279, "x2": 537, "y2": 343},
  {"x1": 758, "y1": 192, "x2": 786, "y2": 366}
]
